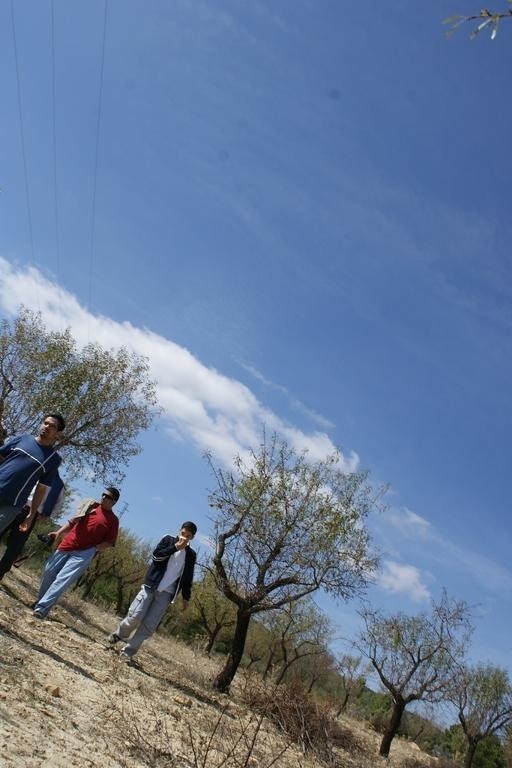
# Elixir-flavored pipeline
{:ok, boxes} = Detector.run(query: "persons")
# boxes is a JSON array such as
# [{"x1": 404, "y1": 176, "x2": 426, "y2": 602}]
[
  {"x1": 0, "y1": 413, "x2": 67, "y2": 535},
  {"x1": 106, "y1": 521, "x2": 197, "y2": 667},
  {"x1": 0, "y1": 469, "x2": 66, "y2": 582},
  {"x1": 30, "y1": 485, "x2": 120, "y2": 619}
]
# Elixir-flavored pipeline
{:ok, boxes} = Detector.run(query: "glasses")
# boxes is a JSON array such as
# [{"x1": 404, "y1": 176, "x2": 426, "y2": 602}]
[{"x1": 101, "y1": 492, "x2": 115, "y2": 500}]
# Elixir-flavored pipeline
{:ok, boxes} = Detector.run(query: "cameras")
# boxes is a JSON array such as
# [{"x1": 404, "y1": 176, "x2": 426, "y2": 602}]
[{"x1": 36, "y1": 533, "x2": 57, "y2": 545}]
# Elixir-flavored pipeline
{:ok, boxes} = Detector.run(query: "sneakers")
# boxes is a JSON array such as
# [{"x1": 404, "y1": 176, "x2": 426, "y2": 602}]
[
  {"x1": 28, "y1": 600, "x2": 45, "y2": 619},
  {"x1": 109, "y1": 633, "x2": 136, "y2": 667}
]
